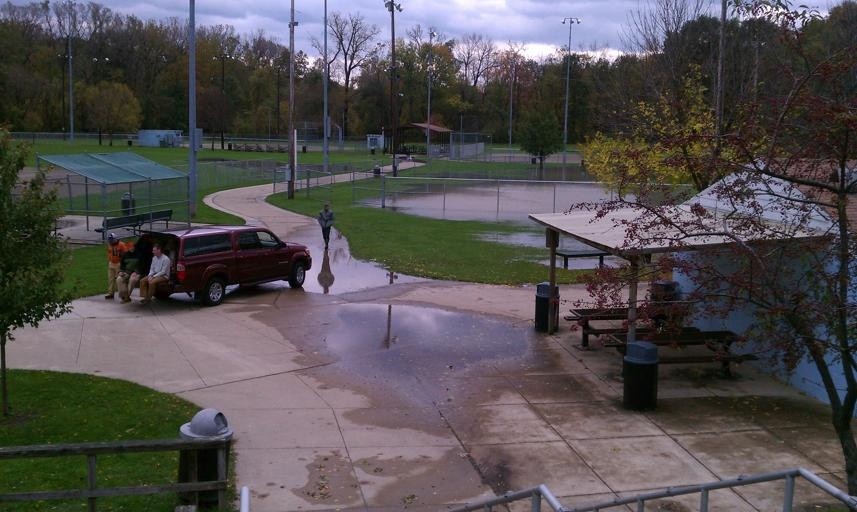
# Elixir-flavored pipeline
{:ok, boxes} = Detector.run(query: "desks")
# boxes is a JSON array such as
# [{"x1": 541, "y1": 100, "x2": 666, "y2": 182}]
[{"x1": 614, "y1": 329, "x2": 742, "y2": 380}]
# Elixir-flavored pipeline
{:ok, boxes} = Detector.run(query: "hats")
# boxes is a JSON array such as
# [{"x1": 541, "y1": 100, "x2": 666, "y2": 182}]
[{"x1": 108, "y1": 233, "x2": 116, "y2": 240}]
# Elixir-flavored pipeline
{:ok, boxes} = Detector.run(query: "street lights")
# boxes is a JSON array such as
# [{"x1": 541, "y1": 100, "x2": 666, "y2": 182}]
[
  {"x1": 385, "y1": 54, "x2": 400, "y2": 156},
  {"x1": 425, "y1": 28, "x2": 436, "y2": 161},
  {"x1": 506, "y1": 59, "x2": 516, "y2": 149},
  {"x1": 560, "y1": 16, "x2": 581, "y2": 183},
  {"x1": 92, "y1": 57, "x2": 109, "y2": 145},
  {"x1": 57, "y1": 54, "x2": 73, "y2": 142},
  {"x1": 276, "y1": 65, "x2": 284, "y2": 138},
  {"x1": 383, "y1": 1, "x2": 405, "y2": 177},
  {"x1": 212, "y1": 53, "x2": 236, "y2": 150}
]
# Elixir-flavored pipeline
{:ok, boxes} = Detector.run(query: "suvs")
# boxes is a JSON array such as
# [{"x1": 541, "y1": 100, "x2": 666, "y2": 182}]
[{"x1": 121, "y1": 224, "x2": 312, "y2": 307}]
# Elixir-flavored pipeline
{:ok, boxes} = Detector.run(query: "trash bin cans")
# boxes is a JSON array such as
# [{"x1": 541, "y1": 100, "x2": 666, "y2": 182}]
[
  {"x1": 622, "y1": 341, "x2": 660, "y2": 411},
  {"x1": 177, "y1": 409, "x2": 233, "y2": 507},
  {"x1": 535, "y1": 282, "x2": 560, "y2": 332},
  {"x1": 121, "y1": 192, "x2": 136, "y2": 215},
  {"x1": 373, "y1": 164, "x2": 381, "y2": 177}
]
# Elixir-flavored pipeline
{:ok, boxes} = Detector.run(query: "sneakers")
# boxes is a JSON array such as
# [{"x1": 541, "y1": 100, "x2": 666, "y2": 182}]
[{"x1": 105, "y1": 295, "x2": 151, "y2": 304}]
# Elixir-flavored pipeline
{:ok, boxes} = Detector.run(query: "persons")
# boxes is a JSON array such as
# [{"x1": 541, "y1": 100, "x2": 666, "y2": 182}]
[
  {"x1": 318, "y1": 249, "x2": 334, "y2": 294},
  {"x1": 139, "y1": 245, "x2": 171, "y2": 304},
  {"x1": 319, "y1": 205, "x2": 333, "y2": 250},
  {"x1": 117, "y1": 243, "x2": 143, "y2": 304},
  {"x1": 105, "y1": 234, "x2": 125, "y2": 298}
]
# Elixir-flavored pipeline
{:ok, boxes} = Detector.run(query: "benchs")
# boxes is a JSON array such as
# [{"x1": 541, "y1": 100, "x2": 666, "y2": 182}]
[
  {"x1": 564, "y1": 306, "x2": 699, "y2": 347},
  {"x1": 132, "y1": 209, "x2": 176, "y2": 232},
  {"x1": 93, "y1": 213, "x2": 144, "y2": 242},
  {"x1": 658, "y1": 354, "x2": 755, "y2": 376}
]
